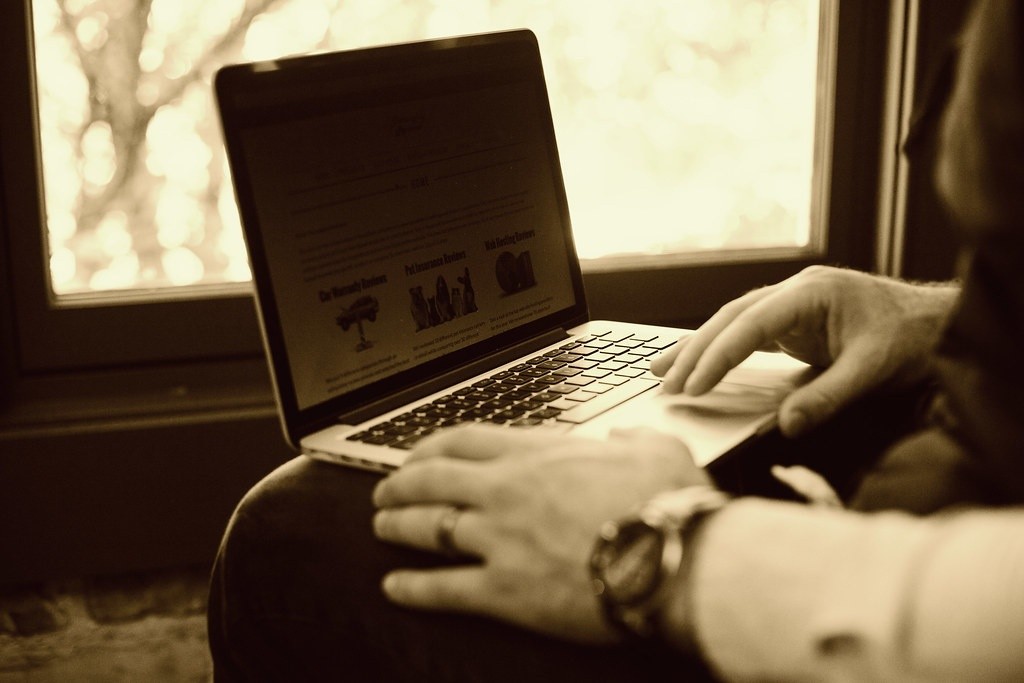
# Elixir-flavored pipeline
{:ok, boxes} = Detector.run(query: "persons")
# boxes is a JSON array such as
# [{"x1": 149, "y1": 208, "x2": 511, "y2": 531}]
[{"x1": 373, "y1": 0, "x2": 1024, "y2": 683}]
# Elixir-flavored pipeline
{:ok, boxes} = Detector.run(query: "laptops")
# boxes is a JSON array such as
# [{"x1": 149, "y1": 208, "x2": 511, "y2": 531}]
[{"x1": 208, "y1": 28, "x2": 824, "y2": 476}]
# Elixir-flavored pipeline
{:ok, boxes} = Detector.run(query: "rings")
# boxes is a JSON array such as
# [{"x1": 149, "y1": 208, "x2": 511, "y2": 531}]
[{"x1": 438, "y1": 504, "x2": 469, "y2": 558}]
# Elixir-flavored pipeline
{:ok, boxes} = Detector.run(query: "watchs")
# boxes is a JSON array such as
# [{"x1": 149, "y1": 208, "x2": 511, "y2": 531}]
[{"x1": 592, "y1": 486, "x2": 731, "y2": 661}]
